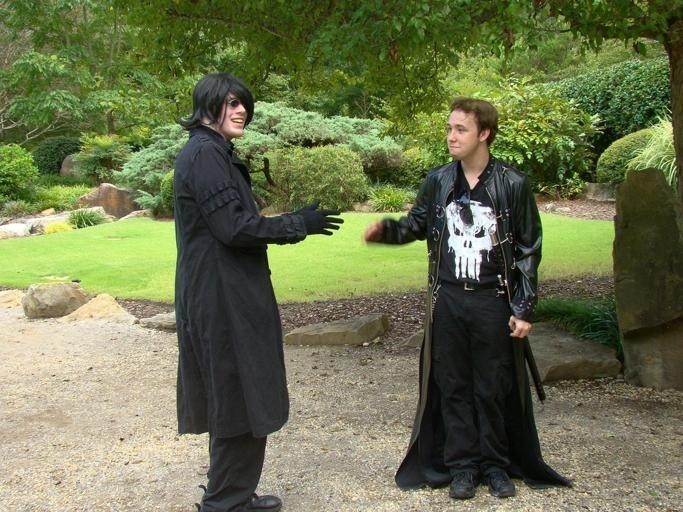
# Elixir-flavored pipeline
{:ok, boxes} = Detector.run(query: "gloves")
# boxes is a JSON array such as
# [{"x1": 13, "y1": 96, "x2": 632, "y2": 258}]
[{"x1": 296, "y1": 200, "x2": 345, "y2": 236}]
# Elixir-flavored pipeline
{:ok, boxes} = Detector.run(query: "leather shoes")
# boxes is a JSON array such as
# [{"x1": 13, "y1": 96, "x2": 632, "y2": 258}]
[{"x1": 246, "y1": 492, "x2": 282, "y2": 512}]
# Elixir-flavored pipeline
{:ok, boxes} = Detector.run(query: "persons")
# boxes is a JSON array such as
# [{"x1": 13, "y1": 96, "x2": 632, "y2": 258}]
[
  {"x1": 171, "y1": 73, "x2": 345, "y2": 512},
  {"x1": 362, "y1": 97, "x2": 574, "y2": 498}
]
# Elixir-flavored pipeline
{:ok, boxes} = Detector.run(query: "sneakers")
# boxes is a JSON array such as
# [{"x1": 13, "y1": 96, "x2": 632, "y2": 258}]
[
  {"x1": 449, "y1": 468, "x2": 479, "y2": 499},
  {"x1": 485, "y1": 469, "x2": 517, "y2": 498}
]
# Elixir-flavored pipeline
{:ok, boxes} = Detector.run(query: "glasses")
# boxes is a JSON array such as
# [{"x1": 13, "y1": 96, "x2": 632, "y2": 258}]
[
  {"x1": 459, "y1": 189, "x2": 474, "y2": 228},
  {"x1": 226, "y1": 99, "x2": 241, "y2": 107}
]
